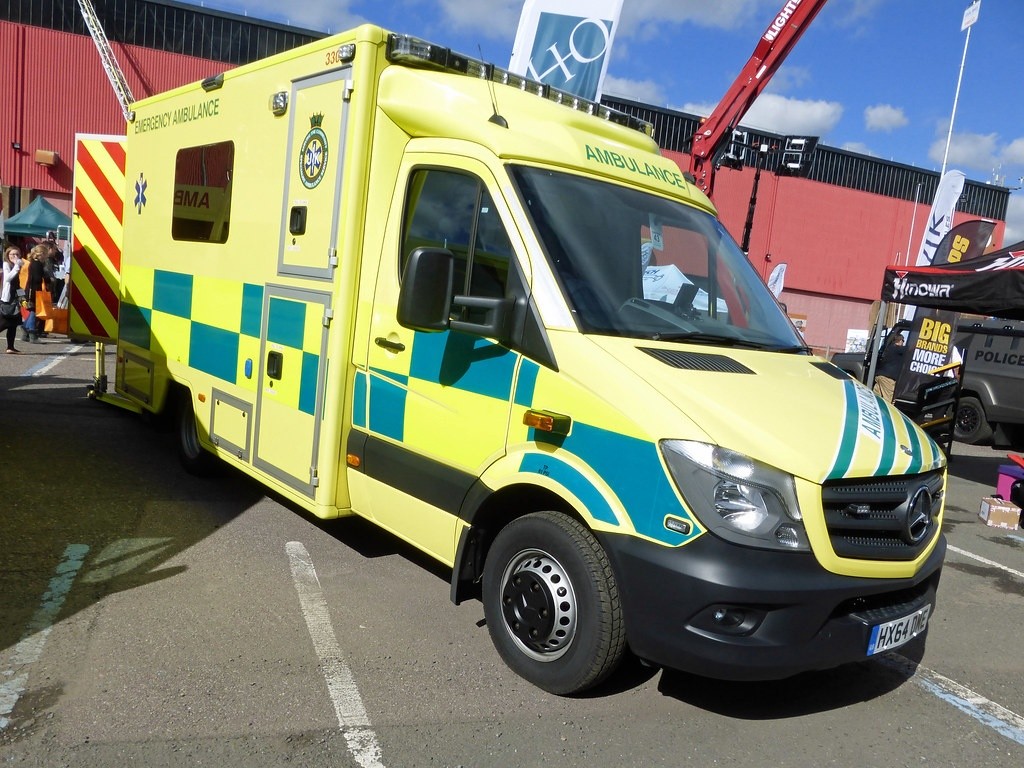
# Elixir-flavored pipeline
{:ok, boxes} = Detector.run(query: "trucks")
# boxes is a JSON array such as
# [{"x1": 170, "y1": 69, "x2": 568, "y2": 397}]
[{"x1": 830, "y1": 319, "x2": 1024, "y2": 446}]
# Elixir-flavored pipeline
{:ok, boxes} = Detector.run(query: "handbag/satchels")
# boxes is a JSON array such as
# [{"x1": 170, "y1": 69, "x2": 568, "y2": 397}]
[
  {"x1": 36, "y1": 290, "x2": 54, "y2": 319},
  {"x1": 0, "y1": 304, "x2": 16, "y2": 316},
  {"x1": 45, "y1": 308, "x2": 68, "y2": 335}
]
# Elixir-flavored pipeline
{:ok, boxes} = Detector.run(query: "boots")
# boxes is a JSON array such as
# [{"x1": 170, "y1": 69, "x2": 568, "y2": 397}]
[{"x1": 20, "y1": 326, "x2": 47, "y2": 344}]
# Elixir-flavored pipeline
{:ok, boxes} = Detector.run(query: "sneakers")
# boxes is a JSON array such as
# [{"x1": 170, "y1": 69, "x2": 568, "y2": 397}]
[{"x1": 6, "y1": 348, "x2": 25, "y2": 355}]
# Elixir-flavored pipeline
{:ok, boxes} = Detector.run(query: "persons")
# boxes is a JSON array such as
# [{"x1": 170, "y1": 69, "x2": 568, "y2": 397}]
[
  {"x1": 873, "y1": 333, "x2": 907, "y2": 402},
  {"x1": 0, "y1": 240, "x2": 70, "y2": 353}
]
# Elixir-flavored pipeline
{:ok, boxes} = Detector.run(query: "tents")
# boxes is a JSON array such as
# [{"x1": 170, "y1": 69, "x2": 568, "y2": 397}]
[
  {"x1": 642, "y1": 264, "x2": 728, "y2": 315},
  {"x1": 5, "y1": 194, "x2": 71, "y2": 241},
  {"x1": 867, "y1": 240, "x2": 1024, "y2": 391}
]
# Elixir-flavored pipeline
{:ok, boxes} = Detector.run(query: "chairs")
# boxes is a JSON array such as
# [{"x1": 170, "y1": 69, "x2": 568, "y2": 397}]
[
  {"x1": 559, "y1": 199, "x2": 626, "y2": 307},
  {"x1": 432, "y1": 204, "x2": 507, "y2": 297}
]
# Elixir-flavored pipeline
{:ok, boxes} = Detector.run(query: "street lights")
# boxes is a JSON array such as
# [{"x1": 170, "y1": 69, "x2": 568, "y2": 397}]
[{"x1": 715, "y1": 135, "x2": 762, "y2": 255}]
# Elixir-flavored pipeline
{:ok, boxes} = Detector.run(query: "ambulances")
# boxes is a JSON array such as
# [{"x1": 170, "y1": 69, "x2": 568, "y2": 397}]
[{"x1": 66, "y1": 22, "x2": 947, "y2": 699}]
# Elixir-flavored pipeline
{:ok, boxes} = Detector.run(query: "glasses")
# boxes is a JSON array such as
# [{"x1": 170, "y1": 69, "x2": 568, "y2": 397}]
[
  {"x1": 9, "y1": 253, "x2": 19, "y2": 256},
  {"x1": 902, "y1": 339, "x2": 904, "y2": 342}
]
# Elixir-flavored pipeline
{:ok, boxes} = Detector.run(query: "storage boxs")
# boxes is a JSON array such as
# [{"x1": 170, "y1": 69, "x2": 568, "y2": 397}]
[
  {"x1": 979, "y1": 497, "x2": 1022, "y2": 531},
  {"x1": 996, "y1": 464, "x2": 1024, "y2": 501}
]
